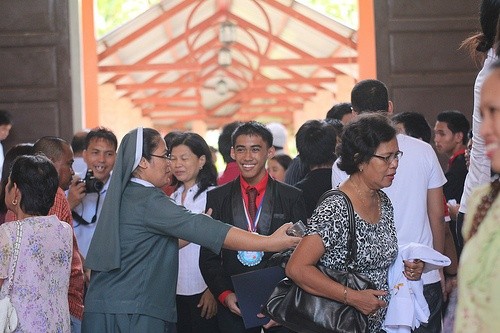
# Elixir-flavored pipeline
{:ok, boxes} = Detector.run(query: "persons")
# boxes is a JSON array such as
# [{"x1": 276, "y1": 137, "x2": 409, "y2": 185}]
[
  {"x1": 453, "y1": 0, "x2": 500, "y2": 261},
  {"x1": 450, "y1": 57, "x2": 500, "y2": 333},
  {"x1": 0, "y1": 79, "x2": 473, "y2": 333}
]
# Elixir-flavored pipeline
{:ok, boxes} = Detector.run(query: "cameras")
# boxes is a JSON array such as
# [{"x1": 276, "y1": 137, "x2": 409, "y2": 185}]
[
  {"x1": 286, "y1": 219, "x2": 307, "y2": 237},
  {"x1": 77, "y1": 169, "x2": 102, "y2": 193}
]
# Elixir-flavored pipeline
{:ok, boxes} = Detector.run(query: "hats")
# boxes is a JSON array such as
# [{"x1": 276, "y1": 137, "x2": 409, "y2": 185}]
[{"x1": 265, "y1": 123, "x2": 287, "y2": 147}]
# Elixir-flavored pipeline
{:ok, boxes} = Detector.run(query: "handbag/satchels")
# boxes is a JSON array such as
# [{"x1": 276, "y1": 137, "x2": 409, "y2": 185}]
[
  {"x1": 264, "y1": 189, "x2": 378, "y2": 333},
  {"x1": 0, "y1": 221, "x2": 22, "y2": 333}
]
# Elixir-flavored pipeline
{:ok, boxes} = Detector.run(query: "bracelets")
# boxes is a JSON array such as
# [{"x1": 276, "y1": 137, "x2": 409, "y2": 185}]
[{"x1": 343, "y1": 285, "x2": 348, "y2": 306}]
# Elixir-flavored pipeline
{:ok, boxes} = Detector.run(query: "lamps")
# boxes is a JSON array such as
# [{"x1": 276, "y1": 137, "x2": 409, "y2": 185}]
[{"x1": 217, "y1": 15, "x2": 233, "y2": 95}]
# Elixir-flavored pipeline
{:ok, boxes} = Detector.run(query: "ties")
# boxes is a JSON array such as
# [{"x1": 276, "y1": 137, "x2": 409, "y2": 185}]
[{"x1": 246, "y1": 188, "x2": 260, "y2": 224}]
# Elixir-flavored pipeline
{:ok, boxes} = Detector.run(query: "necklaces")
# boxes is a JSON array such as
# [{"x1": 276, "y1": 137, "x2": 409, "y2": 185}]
[
  {"x1": 468, "y1": 180, "x2": 500, "y2": 239},
  {"x1": 349, "y1": 176, "x2": 380, "y2": 223}
]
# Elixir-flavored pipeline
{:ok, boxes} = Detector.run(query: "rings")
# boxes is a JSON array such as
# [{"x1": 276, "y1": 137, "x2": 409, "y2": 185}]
[
  {"x1": 410, "y1": 268, "x2": 414, "y2": 273},
  {"x1": 410, "y1": 272, "x2": 414, "y2": 277}
]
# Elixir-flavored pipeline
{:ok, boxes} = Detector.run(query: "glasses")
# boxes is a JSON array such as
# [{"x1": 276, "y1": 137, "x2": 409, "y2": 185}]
[
  {"x1": 372, "y1": 150, "x2": 403, "y2": 165},
  {"x1": 152, "y1": 152, "x2": 170, "y2": 159}
]
